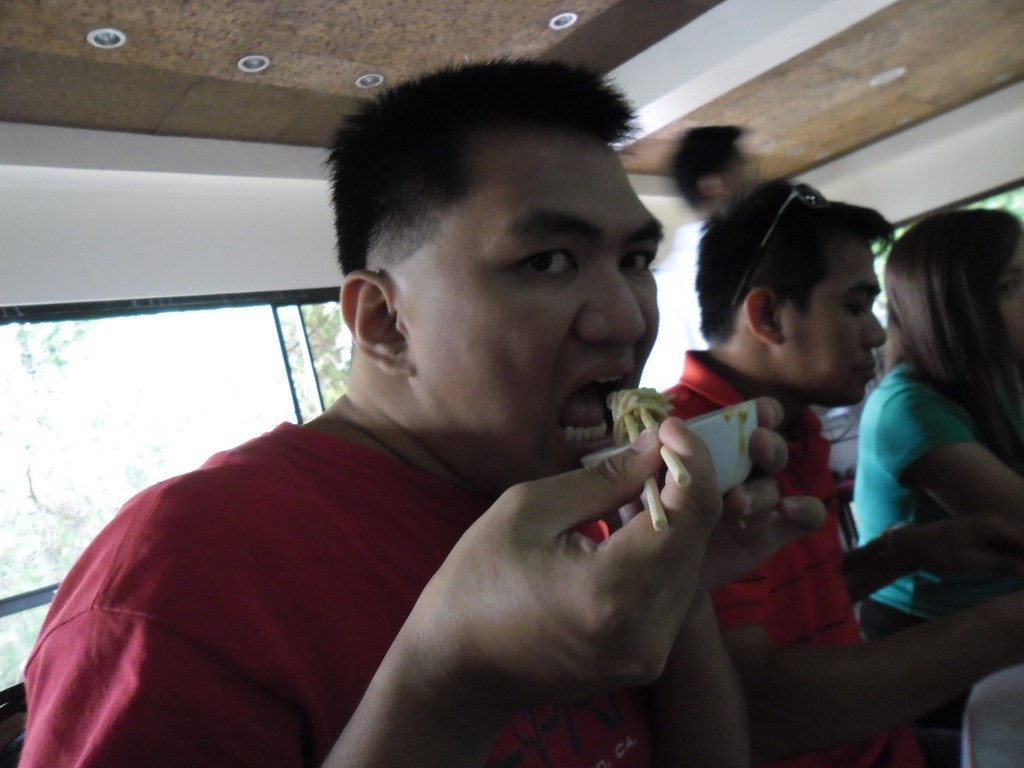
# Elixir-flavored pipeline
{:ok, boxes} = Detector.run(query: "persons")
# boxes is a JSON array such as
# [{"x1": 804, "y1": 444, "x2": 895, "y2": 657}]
[
  {"x1": 14, "y1": 60, "x2": 827, "y2": 766},
  {"x1": 856, "y1": 207, "x2": 1024, "y2": 724},
  {"x1": 657, "y1": 125, "x2": 1024, "y2": 768}
]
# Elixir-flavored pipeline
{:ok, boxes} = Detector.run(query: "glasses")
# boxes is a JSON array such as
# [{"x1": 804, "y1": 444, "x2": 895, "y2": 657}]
[{"x1": 730, "y1": 183, "x2": 831, "y2": 307}]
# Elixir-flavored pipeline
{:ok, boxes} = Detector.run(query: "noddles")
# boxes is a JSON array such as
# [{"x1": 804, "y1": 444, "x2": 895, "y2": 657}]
[{"x1": 609, "y1": 387, "x2": 676, "y2": 448}]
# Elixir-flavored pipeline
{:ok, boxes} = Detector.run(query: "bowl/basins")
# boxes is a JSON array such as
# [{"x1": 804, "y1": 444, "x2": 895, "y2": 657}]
[{"x1": 580, "y1": 398, "x2": 759, "y2": 495}]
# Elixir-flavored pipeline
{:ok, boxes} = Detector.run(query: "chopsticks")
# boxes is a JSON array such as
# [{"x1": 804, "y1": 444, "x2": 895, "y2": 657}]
[{"x1": 620, "y1": 409, "x2": 691, "y2": 530}]
